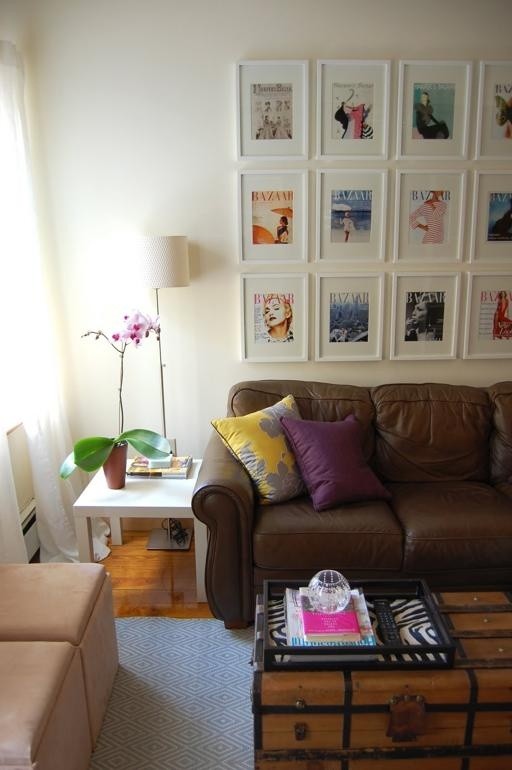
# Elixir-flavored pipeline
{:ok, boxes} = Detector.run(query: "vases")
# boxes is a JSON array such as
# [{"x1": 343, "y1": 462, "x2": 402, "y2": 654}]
[{"x1": 103, "y1": 436, "x2": 128, "y2": 490}]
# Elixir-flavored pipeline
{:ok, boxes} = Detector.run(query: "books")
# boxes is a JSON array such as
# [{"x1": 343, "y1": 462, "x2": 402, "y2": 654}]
[
  {"x1": 126, "y1": 453, "x2": 192, "y2": 480},
  {"x1": 300, "y1": 587, "x2": 362, "y2": 642},
  {"x1": 285, "y1": 588, "x2": 376, "y2": 647}
]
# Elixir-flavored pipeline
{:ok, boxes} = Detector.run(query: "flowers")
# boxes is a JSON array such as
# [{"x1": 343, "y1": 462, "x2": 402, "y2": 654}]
[{"x1": 61, "y1": 312, "x2": 172, "y2": 472}]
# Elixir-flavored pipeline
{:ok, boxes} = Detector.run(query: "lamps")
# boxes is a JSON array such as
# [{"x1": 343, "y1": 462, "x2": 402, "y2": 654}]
[{"x1": 141, "y1": 235, "x2": 194, "y2": 551}]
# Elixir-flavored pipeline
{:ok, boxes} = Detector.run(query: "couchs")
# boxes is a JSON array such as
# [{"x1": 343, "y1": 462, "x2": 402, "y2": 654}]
[{"x1": 191, "y1": 379, "x2": 512, "y2": 629}]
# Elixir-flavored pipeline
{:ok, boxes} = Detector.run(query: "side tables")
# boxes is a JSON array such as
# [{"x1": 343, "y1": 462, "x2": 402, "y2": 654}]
[{"x1": 71, "y1": 459, "x2": 208, "y2": 604}]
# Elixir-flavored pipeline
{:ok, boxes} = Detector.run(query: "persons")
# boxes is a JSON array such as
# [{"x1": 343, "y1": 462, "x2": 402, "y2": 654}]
[
  {"x1": 409, "y1": 191, "x2": 447, "y2": 244},
  {"x1": 257, "y1": 296, "x2": 293, "y2": 343},
  {"x1": 414, "y1": 92, "x2": 448, "y2": 140},
  {"x1": 258, "y1": 115, "x2": 274, "y2": 139},
  {"x1": 278, "y1": 217, "x2": 288, "y2": 244},
  {"x1": 274, "y1": 115, "x2": 289, "y2": 139},
  {"x1": 339, "y1": 212, "x2": 356, "y2": 242},
  {"x1": 492, "y1": 289, "x2": 512, "y2": 339},
  {"x1": 404, "y1": 292, "x2": 439, "y2": 341}
]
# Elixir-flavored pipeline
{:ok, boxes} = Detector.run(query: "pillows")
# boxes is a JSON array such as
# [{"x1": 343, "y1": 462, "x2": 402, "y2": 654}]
[
  {"x1": 278, "y1": 413, "x2": 392, "y2": 511},
  {"x1": 210, "y1": 394, "x2": 302, "y2": 506}
]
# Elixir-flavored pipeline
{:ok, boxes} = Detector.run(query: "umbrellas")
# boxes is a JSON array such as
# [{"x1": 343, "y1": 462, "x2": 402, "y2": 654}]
[
  {"x1": 271, "y1": 208, "x2": 292, "y2": 217},
  {"x1": 332, "y1": 204, "x2": 352, "y2": 211}
]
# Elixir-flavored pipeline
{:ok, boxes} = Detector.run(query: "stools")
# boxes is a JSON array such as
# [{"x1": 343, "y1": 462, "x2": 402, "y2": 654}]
[{"x1": 0, "y1": 564, "x2": 120, "y2": 770}]
